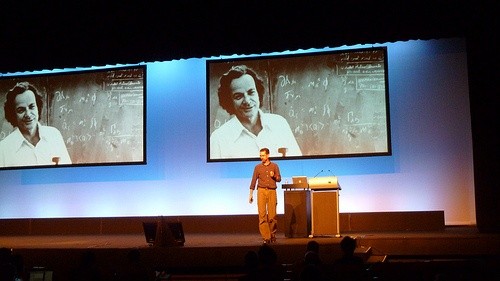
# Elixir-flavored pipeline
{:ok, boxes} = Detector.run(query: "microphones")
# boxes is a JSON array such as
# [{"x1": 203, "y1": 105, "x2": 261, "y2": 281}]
[
  {"x1": 314, "y1": 170, "x2": 323, "y2": 178},
  {"x1": 328, "y1": 170, "x2": 335, "y2": 176}
]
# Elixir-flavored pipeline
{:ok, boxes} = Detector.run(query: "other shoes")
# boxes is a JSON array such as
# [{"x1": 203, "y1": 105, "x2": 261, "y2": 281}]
[
  {"x1": 270, "y1": 236, "x2": 277, "y2": 243},
  {"x1": 262, "y1": 238, "x2": 270, "y2": 245}
]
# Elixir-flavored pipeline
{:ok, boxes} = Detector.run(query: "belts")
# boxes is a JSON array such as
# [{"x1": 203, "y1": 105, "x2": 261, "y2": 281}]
[{"x1": 257, "y1": 185, "x2": 277, "y2": 190}]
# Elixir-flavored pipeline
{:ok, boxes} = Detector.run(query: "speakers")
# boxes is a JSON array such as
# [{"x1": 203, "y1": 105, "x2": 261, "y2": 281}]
[{"x1": 141, "y1": 222, "x2": 186, "y2": 246}]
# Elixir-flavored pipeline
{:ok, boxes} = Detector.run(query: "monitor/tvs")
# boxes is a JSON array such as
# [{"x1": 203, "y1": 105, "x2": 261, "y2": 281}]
[{"x1": 292, "y1": 177, "x2": 307, "y2": 184}]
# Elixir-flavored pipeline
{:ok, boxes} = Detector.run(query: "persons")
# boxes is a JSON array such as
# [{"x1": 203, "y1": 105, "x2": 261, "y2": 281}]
[
  {"x1": 119, "y1": 249, "x2": 150, "y2": 281},
  {"x1": 238, "y1": 236, "x2": 371, "y2": 281},
  {"x1": 0, "y1": 247, "x2": 25, "y2": 281},
  {"x1": 0, "y1": 82, "x2": 72, "y2": 167},
  {"x1": 249, "y1": 148, "x2": 281, "y2": 245},
  {"x1": 66, "y1": 251, "x2": 101, "y2": 281},
  {"x1": 209, "y1": 65, "x2": 303, "y2": 160}
]
